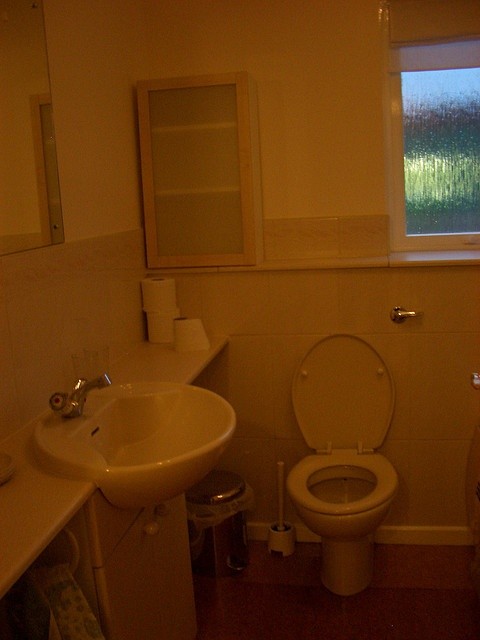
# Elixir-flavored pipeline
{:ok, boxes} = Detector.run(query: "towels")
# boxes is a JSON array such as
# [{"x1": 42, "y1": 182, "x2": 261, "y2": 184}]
[{"x1": 29, "y1": 564, "x2": 105, "y2": 640}]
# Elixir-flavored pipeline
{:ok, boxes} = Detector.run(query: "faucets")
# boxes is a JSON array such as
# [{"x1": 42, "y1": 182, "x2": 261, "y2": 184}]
[{"x1": 71, "y1": 374, "x2": 113, "y2": 416}]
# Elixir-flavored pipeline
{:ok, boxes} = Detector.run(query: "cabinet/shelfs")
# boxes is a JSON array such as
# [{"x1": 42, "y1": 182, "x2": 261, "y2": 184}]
[
  {"x1": 46, "y1": 487, "x2": 199, "y2": 640},
  {"x1": 28, "y1": 93, "x2": 64, "y2": 246},
  {"x1": 136, "y1": 70, "x2": 264, "y2": 269}
]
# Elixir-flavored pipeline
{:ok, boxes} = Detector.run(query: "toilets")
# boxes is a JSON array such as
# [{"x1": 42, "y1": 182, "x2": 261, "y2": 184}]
[{"x1": 286, "y1": 333, "x2": 398, "y2": 596}]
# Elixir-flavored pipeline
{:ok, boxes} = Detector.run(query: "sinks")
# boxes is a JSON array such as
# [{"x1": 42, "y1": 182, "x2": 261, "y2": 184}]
[{"x1": 74, "y1": 387, "x2": 236, "y2": 470}]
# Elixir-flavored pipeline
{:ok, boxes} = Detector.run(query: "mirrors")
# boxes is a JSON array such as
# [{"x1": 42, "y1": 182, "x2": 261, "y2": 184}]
[{"x1": 0, "y1": 0, "x2": 66, "y2": 257}]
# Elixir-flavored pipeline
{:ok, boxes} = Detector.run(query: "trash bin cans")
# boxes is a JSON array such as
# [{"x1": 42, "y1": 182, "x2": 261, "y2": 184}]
[{"x1": 185, "y1": 472, "x2": 251, "y2": 575}]
